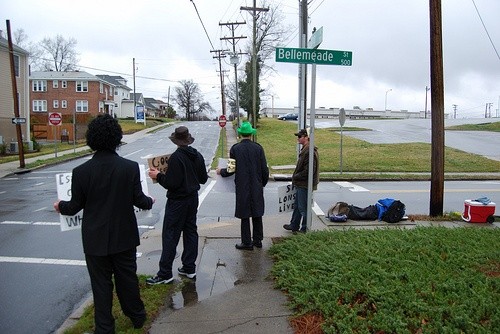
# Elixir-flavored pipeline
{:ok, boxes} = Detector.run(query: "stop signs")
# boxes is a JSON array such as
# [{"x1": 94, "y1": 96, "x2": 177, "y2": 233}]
[
  {"x1": 219, "y1": 115, "x2": 226, "y2": 127},
  {"x1": 49, "y1": 112, "x2": 62, "y2": 126}
]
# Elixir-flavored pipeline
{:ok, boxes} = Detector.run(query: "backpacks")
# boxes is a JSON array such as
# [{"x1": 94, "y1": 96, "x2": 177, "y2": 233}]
[{"x1": 380, "y1": 200, "x2": 407, "y2": 223}]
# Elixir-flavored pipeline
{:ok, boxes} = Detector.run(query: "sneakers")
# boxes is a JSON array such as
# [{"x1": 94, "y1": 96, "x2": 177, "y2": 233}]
[
  {"x1": 145, "y1": 273, "x2": 174, "y2": 285},
  {"x1": 177, "y1": 266, "x2": 197, "y2": 279}
]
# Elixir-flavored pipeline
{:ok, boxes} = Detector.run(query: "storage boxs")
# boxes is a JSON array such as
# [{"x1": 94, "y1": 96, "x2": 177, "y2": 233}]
[{"x1": 464, "y1": 199, "x2": 496, "y2": 224}]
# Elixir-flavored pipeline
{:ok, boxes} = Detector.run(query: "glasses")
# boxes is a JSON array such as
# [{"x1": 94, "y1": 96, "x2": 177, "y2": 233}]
[{"x1": 297, "y1": 135, "x2": 305, "y2": 138}]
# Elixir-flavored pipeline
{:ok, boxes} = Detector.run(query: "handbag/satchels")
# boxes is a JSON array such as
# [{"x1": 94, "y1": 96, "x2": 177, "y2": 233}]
[{"x1": 348, "y1": 204, "x2": 378, "y2": 221}]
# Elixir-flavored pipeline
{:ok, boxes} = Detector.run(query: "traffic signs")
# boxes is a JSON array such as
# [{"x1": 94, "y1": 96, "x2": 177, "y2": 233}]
[{"x1": 11, "y1": 117, "x2": 26, "y2": 124}]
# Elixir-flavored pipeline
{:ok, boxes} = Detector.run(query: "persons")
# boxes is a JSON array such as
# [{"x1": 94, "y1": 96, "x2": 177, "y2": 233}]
[
  {"x1": 53, "y1": 113, "x2": 155, "y2": 334},
  {"x1": 215, "y1": 122, "x2": 269, "y2": 250},
  {"x1": 283, "y1": 129, "x2": 319, "y2": 235},
  {"x1": 146, "y1": 126, "x2": 208, "y2": 286}
]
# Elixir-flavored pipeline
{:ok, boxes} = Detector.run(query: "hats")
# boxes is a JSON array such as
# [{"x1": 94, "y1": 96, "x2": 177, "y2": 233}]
[
  {"x1": 294, "y1": 129, "x2": 310, "y2": 138},
  {"x1": 237, "y1": 121, "x2": 256, "y2": 135},
  {"x1": 168, "y1": 125, "x2": 195, "y2": 146}
]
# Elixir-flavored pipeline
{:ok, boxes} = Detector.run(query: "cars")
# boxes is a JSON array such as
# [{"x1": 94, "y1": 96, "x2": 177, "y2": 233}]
[{"x1": 278, "y1": 113, "x2": 299, "y2": 121}]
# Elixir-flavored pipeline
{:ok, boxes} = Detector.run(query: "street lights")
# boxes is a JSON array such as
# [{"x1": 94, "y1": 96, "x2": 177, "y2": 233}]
[{"x1": 384, "y1": 89, "x2": 393, "y2": 111}]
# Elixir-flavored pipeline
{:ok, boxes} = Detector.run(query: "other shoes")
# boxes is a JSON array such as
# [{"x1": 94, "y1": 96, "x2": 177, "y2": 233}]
[
  {"x1": 235, "y1": 243, "x2": 254, "y2": 251},
  {"x1": 283, "y1": 224, "x2": 300, "y2": 232},
  {"x1": 292, "y1": 230, "x2": 306, "y2": 235},
  {"x1": 251, "y1": 239, "x2": 262, "y2": 248},
  {"x1": 133, "y1": 314, "x2": 147, "y2": 329}
]
